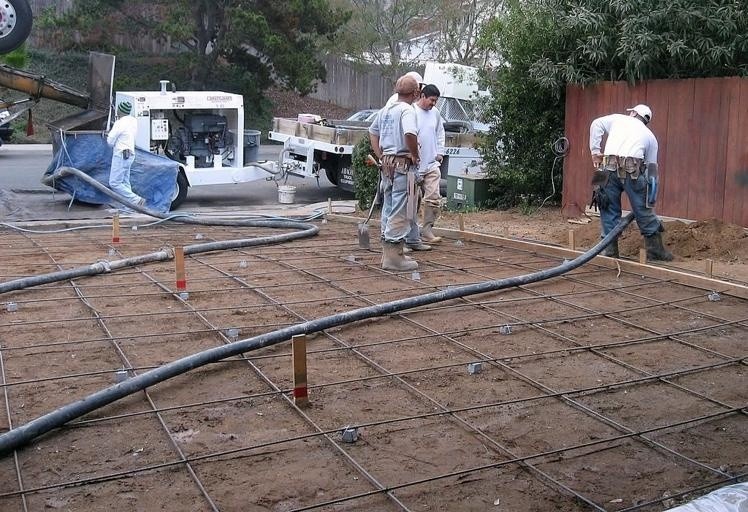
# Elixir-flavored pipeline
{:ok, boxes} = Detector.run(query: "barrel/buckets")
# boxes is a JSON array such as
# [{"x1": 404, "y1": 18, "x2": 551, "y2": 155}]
[{"x1": 278, "y1": 186, "x2": 297, "y2": 204}]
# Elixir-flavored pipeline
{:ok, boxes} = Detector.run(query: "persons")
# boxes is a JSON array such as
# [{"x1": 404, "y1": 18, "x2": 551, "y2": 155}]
[
  {"x1": 369, "y1": 76, "x2": 422, "y2": 275},
  {"x1": 386, "y1": 70, "x2": 432, "y2": 257},
  {"x1": 587, "y1": 103, "x2": 678, "y2": 263},
  {"x1": 408, "y1": 82, "x2": 447, "y2": 246},
  {"x1": 103, "y1": 100, "x2": 149, "y2": 213}
]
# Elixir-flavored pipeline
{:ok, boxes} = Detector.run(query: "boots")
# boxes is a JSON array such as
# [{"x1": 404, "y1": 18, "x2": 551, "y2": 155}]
[
  {"x1": 645, "y1": 234, "x2": 674, "y2": 261},
  {"x1": 381, "y1": 224, "x2": 441, "y2": 270},
  {"x1": 601, "y1": 238, "x2": 619, "y2": 258}
]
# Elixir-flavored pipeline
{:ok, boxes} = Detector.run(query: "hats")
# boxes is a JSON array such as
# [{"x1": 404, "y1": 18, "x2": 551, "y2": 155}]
[
  {"x1": 396, "y1": 72, "x2": 426, "y2": 94},
  {"x1": 626, "y1": 104, "x2": 652, "y2": 122},
  {"x1": 118, "y1": 101, "x2": 131, "y2": 115}
]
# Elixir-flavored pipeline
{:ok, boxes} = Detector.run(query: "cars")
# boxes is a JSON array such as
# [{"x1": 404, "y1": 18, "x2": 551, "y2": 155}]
[
  {"x1": 0, "y1": 1, "x2": 34, "y2": 57},
  {"x1": 345, "y1": 107, "x2": 380, "y2": 123},
  {"x1": 0, "y1": 99, "x2": 14, "y2": 147}
]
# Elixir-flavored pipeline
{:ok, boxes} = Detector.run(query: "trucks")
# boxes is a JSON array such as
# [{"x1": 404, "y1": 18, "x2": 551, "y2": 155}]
[{"x1": 260, "y1": 59, "x2": 502, "y2": 199}]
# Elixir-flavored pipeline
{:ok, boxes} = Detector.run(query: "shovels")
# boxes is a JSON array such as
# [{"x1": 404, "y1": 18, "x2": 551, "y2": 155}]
[{"x1": 358, "y1": 193, "x2": 377, "y2": 248}]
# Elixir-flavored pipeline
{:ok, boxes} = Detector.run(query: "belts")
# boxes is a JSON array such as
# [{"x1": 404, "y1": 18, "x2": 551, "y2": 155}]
[{"x1": 383, "y1": 156, "x2": 412, "y2": 164}]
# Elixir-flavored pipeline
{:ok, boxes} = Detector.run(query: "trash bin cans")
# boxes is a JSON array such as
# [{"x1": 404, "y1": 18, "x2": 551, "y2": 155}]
[{"x1": 228, "y1": 128, "x2": 261, "y2": 166}]
